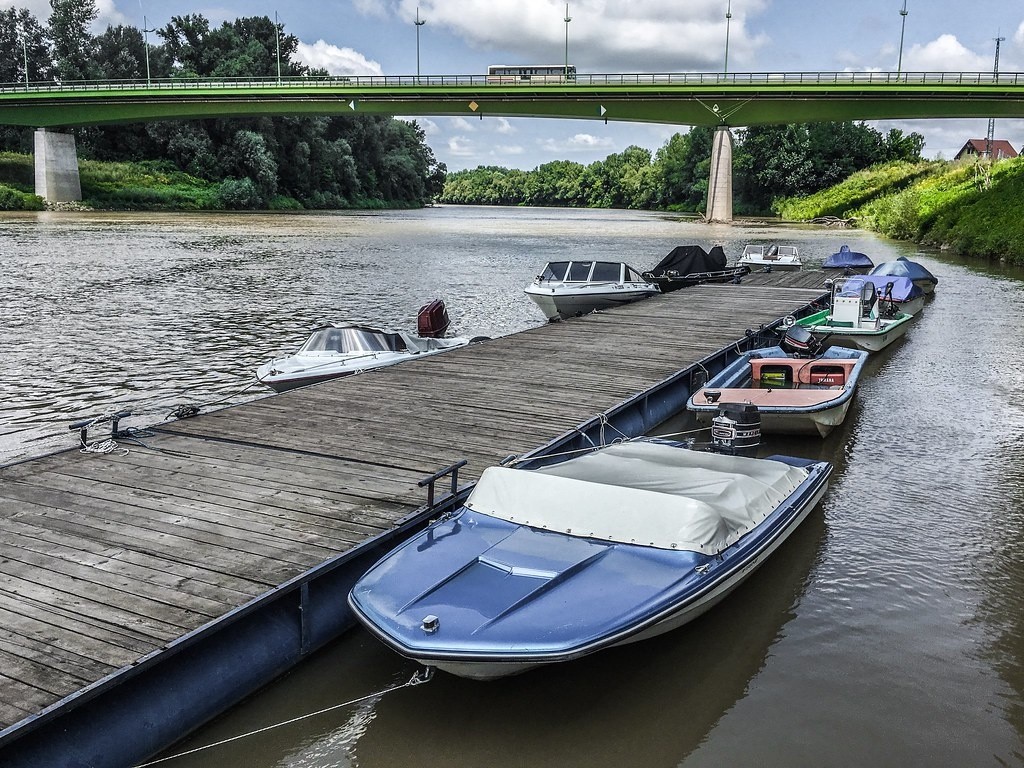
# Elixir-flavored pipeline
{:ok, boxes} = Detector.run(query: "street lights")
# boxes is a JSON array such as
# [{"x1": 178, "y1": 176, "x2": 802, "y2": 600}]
[
  {"x1": 273, "y1": 11, "x2": 283, "y2": 87},
  {"x1": 142, "y1": 15, "x2": 151, "y2": 91},
  {"x1": 413, "y1": 6, "x2": 426, "y2": 83},
  {"x1": 563, "y1": 3, "x2": 571, "y2": 85},
  {"x1": 897, "y1": 0, "x2": 907, "y2": 81},
  {"x1": 722, "y1": 0, "x2": 733, "y2": 82}
]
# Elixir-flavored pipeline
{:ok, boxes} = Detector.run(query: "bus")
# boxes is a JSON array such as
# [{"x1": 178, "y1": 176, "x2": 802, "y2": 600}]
[{"x1": 488, "y1": 65, "x2": 578, "y2": 87}]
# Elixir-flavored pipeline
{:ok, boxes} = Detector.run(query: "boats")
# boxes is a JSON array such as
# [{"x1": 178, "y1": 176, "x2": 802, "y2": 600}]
[
  {"x1": 867, "y1": 256, "x2": 938, "y2": 296},
  {"x1": 738, "y1": 242, "x2": 804, "y2": 275},
  {"x1": 684, "y1": 314, "x2": 870, "y2": 440},
  {"x1": 524, "y1": 257, "x2": 663, "y2": 325},
  {"x1": 642, "y1": 243, "x2": 754, "y2": 294},
  {"x1": 347, "y1": 400, "x2": 837, "y2": 684},
  {"x1": 775, "y1": 274, "x2": 915, "y2": 355},
  {"x1": 822, "y1": 245, "x2": 876, "y2": 280},
  {"x1": 257, "y1": 320, "x2": 489, "y2": 395}
]
